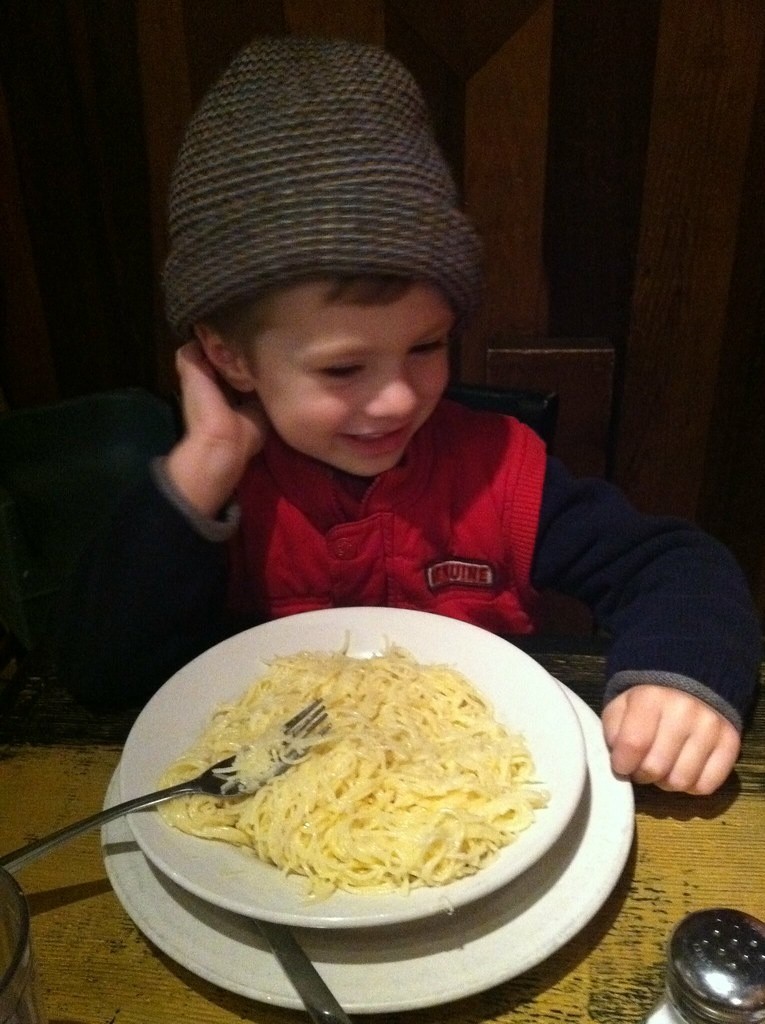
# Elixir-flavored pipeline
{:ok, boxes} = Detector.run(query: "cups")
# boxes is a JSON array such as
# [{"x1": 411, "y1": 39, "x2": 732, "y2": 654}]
[{"x1": 1, "y1": 869, "x2": 30, "y2": 1024}]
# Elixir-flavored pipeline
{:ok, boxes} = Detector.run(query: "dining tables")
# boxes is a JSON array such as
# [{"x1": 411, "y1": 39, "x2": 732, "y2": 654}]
[{"x1": 1, "y1": 634, "x2": 765, "y2": 1023}]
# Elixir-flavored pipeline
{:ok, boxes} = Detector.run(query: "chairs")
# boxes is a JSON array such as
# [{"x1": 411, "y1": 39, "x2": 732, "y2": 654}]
[{"x1": 443, "y1": 328, "x2": 616, "y2": 643}]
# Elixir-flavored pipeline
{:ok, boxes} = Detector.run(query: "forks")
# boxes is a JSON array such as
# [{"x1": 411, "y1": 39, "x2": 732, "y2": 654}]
[{"x1": 0, "y1": 699, "x2": 332, "y2": 873}]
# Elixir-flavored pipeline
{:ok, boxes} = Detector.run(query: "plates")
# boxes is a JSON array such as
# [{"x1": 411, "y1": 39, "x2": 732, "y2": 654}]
[
  {"x1": 645, "y1": 1000, "x2": 685, "y2": 1024},
  {"x1": 101, "y1": 676, "x2": 635, "y2": 1013},
  {"x1": 121, "y1": 607, "x2": 587, "y2": 929}
]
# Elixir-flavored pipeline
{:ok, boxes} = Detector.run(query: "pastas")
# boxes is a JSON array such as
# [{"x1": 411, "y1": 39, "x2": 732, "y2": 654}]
[{"x1": 155, "y1": 631, "x2": 553, "y2": 909}]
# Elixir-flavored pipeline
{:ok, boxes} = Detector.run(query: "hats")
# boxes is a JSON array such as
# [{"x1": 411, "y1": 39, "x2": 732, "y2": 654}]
[{"x1": 158, "y1": 35, "x2": 488, "y2": 324}]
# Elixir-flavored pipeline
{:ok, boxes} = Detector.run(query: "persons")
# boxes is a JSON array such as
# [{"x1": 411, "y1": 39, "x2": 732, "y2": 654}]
[{"x1": 54, "y1": 14, "x2": 763, "y2": 796}]
